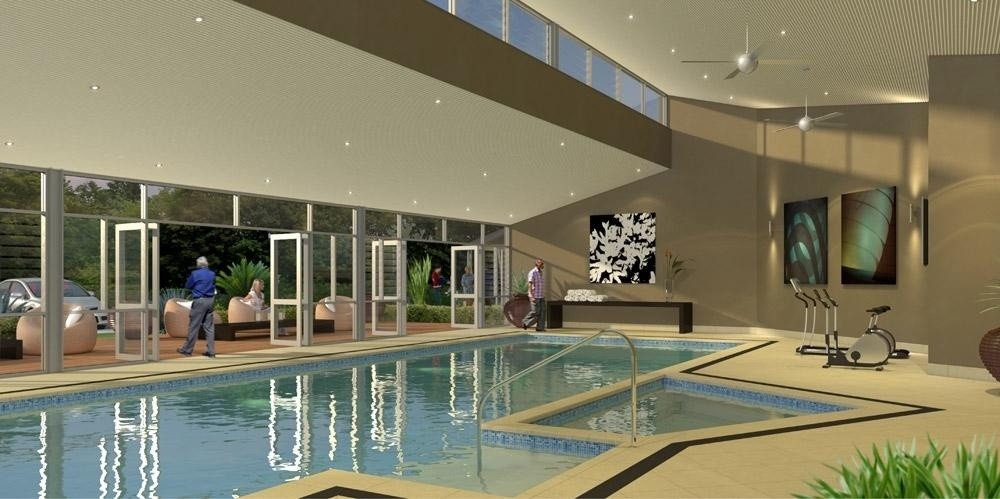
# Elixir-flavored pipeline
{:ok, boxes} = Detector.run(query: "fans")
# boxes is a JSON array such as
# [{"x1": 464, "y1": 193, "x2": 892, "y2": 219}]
[
  {"x1": 678, "y1": 21, "x2": 818, "y2": 85},
  {"x1": 760, "y1": 95, "x2": 850, "y2": 134}
]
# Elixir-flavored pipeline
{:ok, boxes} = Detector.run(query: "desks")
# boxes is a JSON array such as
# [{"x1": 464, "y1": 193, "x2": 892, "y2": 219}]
[
  {"x1": 0, "y1": 338, "x2": 25, "y2": 360},
  {"x1": 195, "y1": 315, "x2": 337, "y2": 339},
  {"x1": 545, "y1": 297, "x2": 694, "y2": 335}
]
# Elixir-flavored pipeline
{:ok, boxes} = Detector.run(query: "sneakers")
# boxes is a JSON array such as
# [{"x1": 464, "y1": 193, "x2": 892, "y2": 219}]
[
  {"x1": 202, "y1": 351, "x2": 215, "y2": 358},
  {"x1": 177, "y1": 348, "x2": 191, "y2": 356}
]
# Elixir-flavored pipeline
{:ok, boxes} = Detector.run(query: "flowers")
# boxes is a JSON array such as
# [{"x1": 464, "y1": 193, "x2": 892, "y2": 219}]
[{"x1": 656, "y1": 244, "x2": 698, "y2": 279}]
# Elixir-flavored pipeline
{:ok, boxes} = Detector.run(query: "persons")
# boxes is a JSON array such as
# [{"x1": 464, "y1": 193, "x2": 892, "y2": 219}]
[
  {"x1": 521, "y1": 258, "x2": 546, "y2": 331},
  {"x1": 431, "y1": 265, "x2": 445, "y2": 304},
  {"x1": 461, "y1": 266, "x2": 474, "y2": 305},
  {"x1": 176, "y1": 256, "x2": 216, "y2": 356},
  {"x1": 244, "y1": 278, "x2": 265, "y2": 306}
]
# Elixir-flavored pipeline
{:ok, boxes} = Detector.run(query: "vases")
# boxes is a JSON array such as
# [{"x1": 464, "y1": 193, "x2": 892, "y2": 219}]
[{"x1": 662, "y1": 275, "x2": 677, "y2": 302}]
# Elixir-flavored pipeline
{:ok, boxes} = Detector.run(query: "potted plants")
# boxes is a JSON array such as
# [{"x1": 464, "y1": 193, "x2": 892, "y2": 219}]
[{"x1": 504, "y1": 270, "x2": 539, "y2": 331}]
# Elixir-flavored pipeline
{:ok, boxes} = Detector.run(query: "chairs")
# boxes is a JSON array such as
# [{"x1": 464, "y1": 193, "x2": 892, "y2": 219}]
[
  {"x1": 224, "y1": 292, "x2": 275, "y2": 325},
  {"x1": 14, "y1": 304, "x2": 100, "y2": 357},
  {"x1": 163, "y1": 296, "x2": 225, "y2": 345},
  {"x1": 108, "y1": 304, "x2": 155, "y2": 342},
  {"x1": 314, "y1": 295, "x2": 354, "y2": 331}
]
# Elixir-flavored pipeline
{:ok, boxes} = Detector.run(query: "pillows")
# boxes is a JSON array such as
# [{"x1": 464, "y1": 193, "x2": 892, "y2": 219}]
[
  {"x1": 240, "y1": 299, "x2": 257, "y2": 309},
  {"x1": 323, "y1": 296, "x2": 336, "y2": 314},
  {"x1": 175, "y1": 299, "x2": 196, "y2": 313},
  {"x1": 64, "y1": 306, "x2": 81, "y2": 328}
]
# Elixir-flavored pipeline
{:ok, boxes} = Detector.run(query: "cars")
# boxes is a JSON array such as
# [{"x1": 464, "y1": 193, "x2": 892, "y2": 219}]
[{"x1": 0, "y1": 275, "x2": 113, "y2": 334}]
